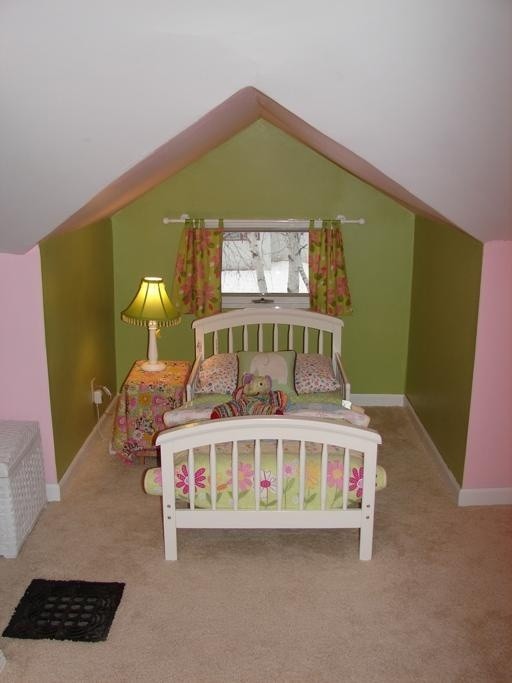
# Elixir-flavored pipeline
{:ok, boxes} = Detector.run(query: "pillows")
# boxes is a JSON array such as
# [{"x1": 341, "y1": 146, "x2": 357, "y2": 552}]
[{"x1": 193, "y1": 349, "x2": 340, "y2": 398}]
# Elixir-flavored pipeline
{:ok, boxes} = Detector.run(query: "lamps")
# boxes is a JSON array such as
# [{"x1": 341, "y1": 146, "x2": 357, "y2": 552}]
[{"x1": 121, "y1": 277, "x2": 181, "y2": 372}]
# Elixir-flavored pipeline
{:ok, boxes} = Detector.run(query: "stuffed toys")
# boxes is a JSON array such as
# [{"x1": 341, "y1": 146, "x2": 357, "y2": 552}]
[{"x1": 210, "y1": 372, "x2": 287, "y2": 420}]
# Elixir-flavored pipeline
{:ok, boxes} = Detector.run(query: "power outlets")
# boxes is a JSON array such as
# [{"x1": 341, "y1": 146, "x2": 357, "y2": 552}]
[{"x1": 91, "y1": 378, "x2": 103, "y2": 405}]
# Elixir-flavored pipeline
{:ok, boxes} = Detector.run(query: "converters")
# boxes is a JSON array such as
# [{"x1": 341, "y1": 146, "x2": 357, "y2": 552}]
[{"x1": 93, "y1": 389, "x2": 103, "y2": 404}]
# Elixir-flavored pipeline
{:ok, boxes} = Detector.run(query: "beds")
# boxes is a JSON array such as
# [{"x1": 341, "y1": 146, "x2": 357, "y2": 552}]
[{"x1": 154, "y1": 308, "x2": 384, "y2": 561}]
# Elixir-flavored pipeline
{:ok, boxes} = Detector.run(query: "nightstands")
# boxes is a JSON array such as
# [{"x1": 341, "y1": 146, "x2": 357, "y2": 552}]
[{"x1": 123, "y1": 359, "x2": 190, "y2": 465}]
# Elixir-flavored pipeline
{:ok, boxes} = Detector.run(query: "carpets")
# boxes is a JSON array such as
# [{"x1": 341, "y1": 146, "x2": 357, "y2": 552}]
[{"x1": 0, "y1": 578, "x2": 126, "y2": 642}]
